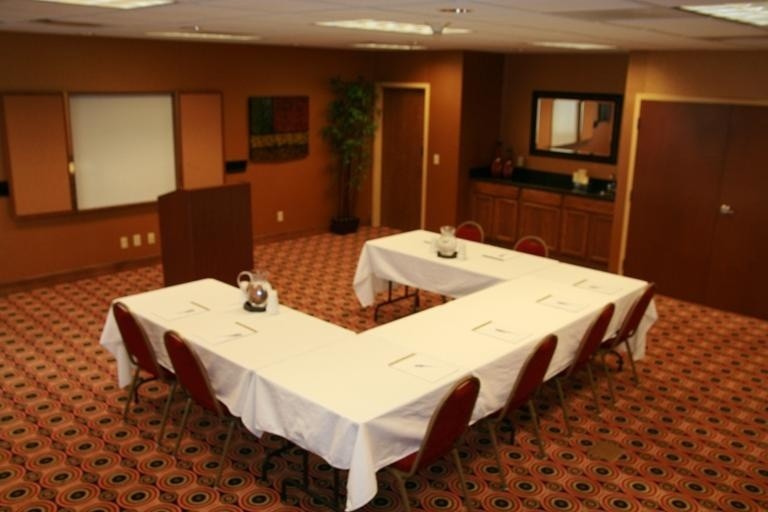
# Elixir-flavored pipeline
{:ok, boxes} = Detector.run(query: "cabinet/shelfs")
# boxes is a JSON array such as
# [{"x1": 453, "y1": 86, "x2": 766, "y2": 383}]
[
  {"x1": 465, "y1": 180, "x2": 519, "y2": 246},
  {"x1": 517, "y1": 188, "x2": 563, "y2": 257},
  {"x1": 560, "y1": 195, "x2": 614, "y2": 263}
]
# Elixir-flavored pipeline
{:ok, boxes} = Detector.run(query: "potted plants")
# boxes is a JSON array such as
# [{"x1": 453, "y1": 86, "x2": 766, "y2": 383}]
[{"x1": 320, "y1": 67, "x2": 388, "y2": 236}]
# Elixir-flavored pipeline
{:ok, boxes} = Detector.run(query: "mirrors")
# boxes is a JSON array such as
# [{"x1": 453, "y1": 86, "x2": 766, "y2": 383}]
[{"x1": 529, "y1": 89, "x2": 624, "y2": 165}]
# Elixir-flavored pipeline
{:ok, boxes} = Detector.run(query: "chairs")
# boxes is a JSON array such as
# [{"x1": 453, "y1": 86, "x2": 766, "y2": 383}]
[{"x1": 98, "y1": 220, "x2": 658, "y2": 511}]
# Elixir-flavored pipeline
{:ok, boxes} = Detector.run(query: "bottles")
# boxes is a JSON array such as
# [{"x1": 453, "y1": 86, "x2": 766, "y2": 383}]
[
  {"x1": 247, "y1": 268, "x2": 270, "y2": 307},
  {"x1": 437, "y1": 226, "x2": 457, "y2": 257}
]
